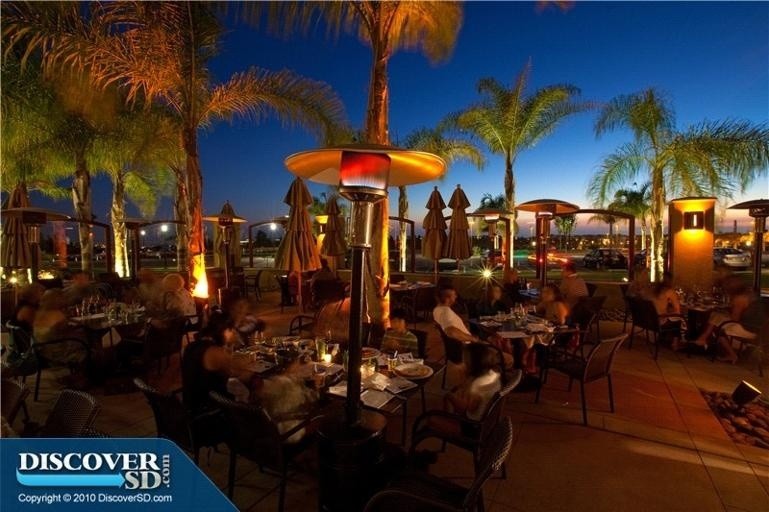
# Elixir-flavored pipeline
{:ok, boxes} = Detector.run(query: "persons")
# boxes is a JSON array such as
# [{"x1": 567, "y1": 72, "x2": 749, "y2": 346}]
[{"x1": 11, "y1": 253, "x2": 764, "y2": 498}]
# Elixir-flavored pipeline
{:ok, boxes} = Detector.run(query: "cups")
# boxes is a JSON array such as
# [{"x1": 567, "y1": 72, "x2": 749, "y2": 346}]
[{"x1": 70, "y1": 297, "x2": 144, "y2": 325}]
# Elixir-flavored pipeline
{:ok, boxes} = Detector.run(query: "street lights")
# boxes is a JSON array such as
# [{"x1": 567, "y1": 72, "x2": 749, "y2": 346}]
[
  {"x1": 270, "y1": 223, "x2": 277, "y2": 256},
  {"x1": 161, "y1": 225, "x2": 169, "y2": 268},
  {"x1": 141, "y1": 231, "x2": 146, "y2": 248}
]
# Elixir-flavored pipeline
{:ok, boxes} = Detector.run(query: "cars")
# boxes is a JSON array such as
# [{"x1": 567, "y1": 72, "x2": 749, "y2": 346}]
[
  {"x1": 477, "y1": 245, "x2": 653, "y2": 272},
  {"x1": 713, "y1": 247, "x2": 752, "y2": 271}
]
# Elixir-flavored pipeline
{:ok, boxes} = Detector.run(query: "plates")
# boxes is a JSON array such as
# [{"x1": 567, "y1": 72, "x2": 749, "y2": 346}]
[
  {"x1": 483, "y1": 313, "x2": 557, "y2": 337},
  {"x1": 302, "y1": 345, "x2": 435, "y2": 395}
]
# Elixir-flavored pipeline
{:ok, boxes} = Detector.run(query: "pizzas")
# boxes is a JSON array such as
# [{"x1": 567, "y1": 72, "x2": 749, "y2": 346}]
[{"x1": 393, "y1": 366, "x2": 429, "y2": 377}]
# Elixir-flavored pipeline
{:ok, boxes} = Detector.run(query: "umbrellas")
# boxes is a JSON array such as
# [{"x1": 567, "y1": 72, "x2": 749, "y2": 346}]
[
  {"x1": 421, "y1": 185, "x2": 449, "y2": 284},
  {"x1": 322, "y1": 196, "x2": 349, "y2": 271},
  {"x1": 274, "y1": 176, "x2": 323, "y2": 315},
  {"x1": 214, "y1": 199, "x2": 241, "y2": 267},
  {"x1": 1, "y1": 181, "x2": 33, "y2": 270},
  {"x1": 443, "y1": 183, "x2": 474, "y2": 260}
]
